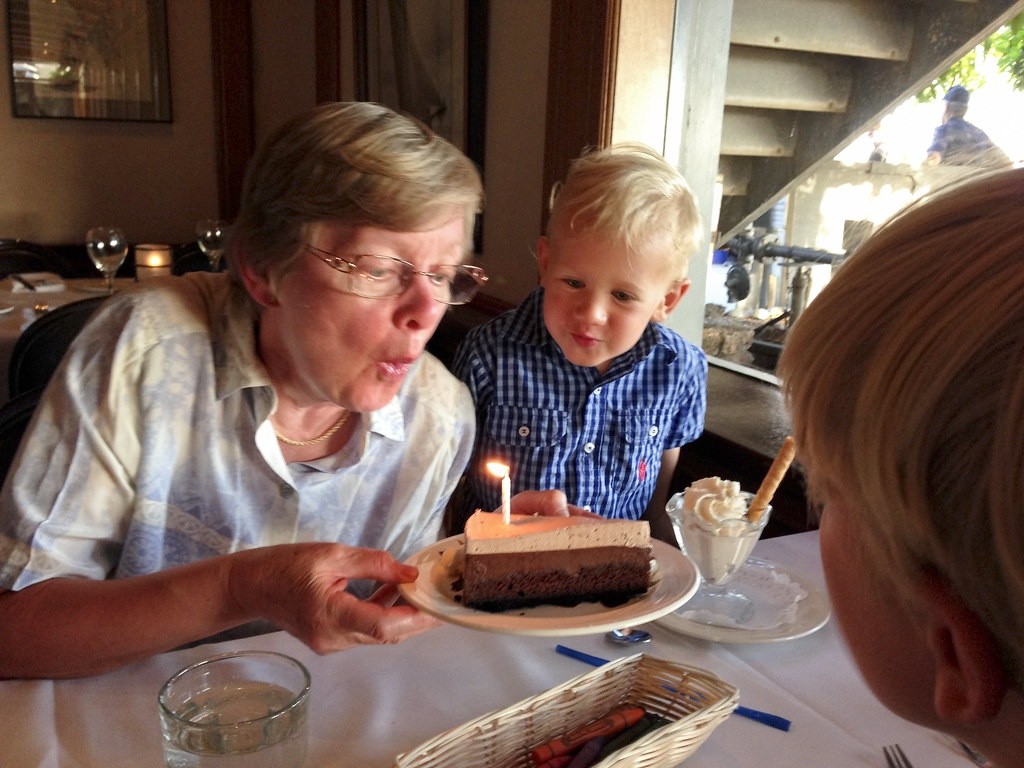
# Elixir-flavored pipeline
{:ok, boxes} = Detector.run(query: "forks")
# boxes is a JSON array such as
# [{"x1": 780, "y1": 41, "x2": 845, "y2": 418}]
[{"x1": 882, "y1": 744, "x2": 913, "y2": 768}]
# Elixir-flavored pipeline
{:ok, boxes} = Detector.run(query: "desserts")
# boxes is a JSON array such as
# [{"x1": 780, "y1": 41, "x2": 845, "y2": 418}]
[{"x1": 463, "y1": 507, "x2": 655, "y2": 608}]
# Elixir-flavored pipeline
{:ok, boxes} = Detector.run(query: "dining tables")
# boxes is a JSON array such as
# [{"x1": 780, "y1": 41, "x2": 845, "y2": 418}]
[
  {"x1": 0, "y1": 520, "x2": 990, "y2": 768},
  {"x1": 0, "y1": 275, "x2": 180, "y2": 412}
]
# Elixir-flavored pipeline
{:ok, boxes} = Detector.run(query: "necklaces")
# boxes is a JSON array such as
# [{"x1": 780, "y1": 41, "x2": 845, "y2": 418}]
[{"x1": 276, "y1": 408, "x2": 351, "y2": 446}]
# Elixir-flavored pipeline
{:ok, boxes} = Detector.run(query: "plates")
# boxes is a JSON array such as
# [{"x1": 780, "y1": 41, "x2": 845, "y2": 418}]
[
  {"x1": 652, "y1": 555, "x2": 832, "y2": 644},
  {"x1": 394, "y1": 533, "x2": 701, "y2": 639}
]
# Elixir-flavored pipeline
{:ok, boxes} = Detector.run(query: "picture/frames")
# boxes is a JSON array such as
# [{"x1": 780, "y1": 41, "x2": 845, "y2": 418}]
[
  {"x1": 351, "y1": 0, "x2": 488, "y2": 258},
  {"x1": 6, "y1": 0, "x2": 174, "y2": 124}
]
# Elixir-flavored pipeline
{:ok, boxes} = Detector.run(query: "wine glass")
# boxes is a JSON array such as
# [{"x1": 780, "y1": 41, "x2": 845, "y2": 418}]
[
  {"x1": 665, "y1": 490, "x2": 772, "y2": 626},
  {"x1": 86, "y1": 224, "x2": 129, "y2": 296},
  {"x1": 196, "y1": 218, "x2": 225, "y2": 273}
]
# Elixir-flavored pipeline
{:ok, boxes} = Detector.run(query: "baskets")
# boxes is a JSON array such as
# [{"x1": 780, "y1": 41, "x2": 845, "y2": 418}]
[{"x1": 392, "y1": 652, "x2": 744, "y2": 768}]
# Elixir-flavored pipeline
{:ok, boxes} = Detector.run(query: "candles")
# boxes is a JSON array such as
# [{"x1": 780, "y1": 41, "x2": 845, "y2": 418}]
[{"x1": 501, "y1": 468, "x2": 512, "y2": 525}]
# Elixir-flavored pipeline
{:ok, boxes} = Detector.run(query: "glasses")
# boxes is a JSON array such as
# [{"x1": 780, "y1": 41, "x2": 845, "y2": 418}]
[{"x1": 292, "y1": 236, "x2": 490, "y2": 305}]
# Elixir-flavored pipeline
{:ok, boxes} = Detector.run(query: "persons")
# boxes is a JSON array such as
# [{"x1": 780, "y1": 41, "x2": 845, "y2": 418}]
[
  {"x1": 774, "y1": 170, "x2": 1023, "y2": 768},
  {"x1": 922, "y1": 82, "x2": 1009, "y2": 171},
  {"x1": 448, "y1": 138, "x2": 708, "y2": 533},
  {"x1": 0, "y1": 101, "x2": 605, "y2": 675}
]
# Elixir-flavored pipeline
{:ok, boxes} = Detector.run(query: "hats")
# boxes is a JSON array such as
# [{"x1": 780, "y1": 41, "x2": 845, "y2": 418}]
[{"x1": 940, "y1": 85, "x2": 970, "y2": 108}]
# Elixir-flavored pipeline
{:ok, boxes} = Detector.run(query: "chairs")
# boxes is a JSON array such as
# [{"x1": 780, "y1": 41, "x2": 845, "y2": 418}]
[{"x1": 0, "y1": 294, "x2": 112, "y2": 491}]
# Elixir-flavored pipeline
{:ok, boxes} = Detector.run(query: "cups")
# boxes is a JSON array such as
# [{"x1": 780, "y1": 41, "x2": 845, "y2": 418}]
[{"x1": 157, "y1": 650, "x2": 313, "y2": 768}]
